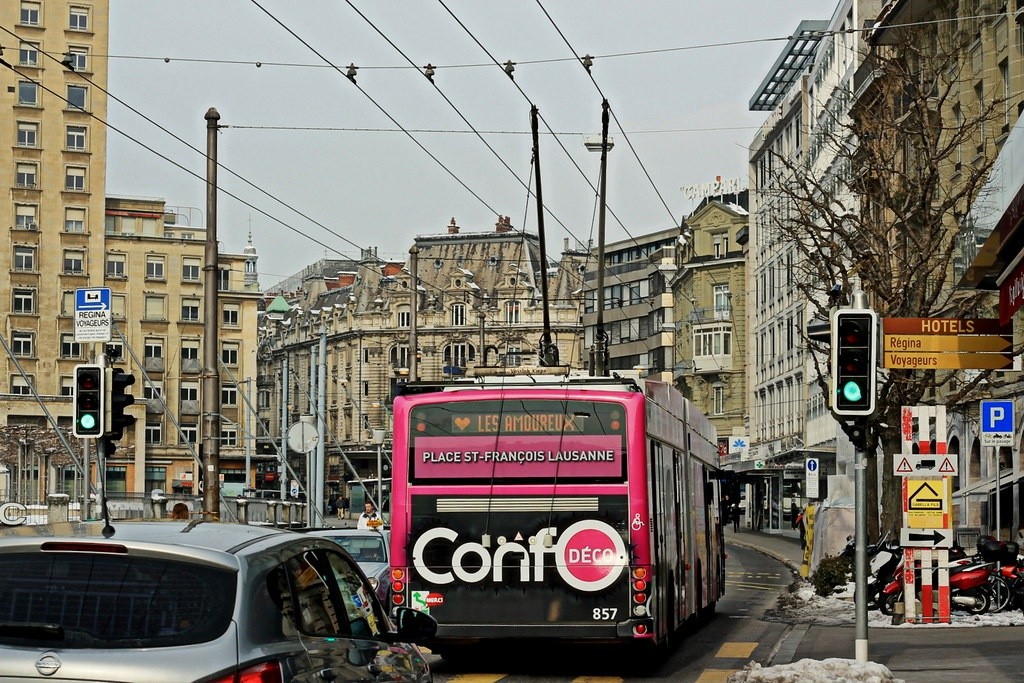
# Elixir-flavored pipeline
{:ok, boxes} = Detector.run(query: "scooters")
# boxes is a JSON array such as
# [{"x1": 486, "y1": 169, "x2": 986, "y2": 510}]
[{"x1": 840, "y1": 528, "x2": 1024, "y2": 616}]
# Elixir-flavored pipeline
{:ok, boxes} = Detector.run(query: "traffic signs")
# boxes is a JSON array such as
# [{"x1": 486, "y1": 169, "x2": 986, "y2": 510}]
[{"x1": 73, "y1": 287, "x2": 112, "y2": 343}]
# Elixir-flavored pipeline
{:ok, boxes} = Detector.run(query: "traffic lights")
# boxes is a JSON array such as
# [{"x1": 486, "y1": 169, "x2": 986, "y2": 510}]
[
  {"x1": 105, "y1": 368, "x2": 135, "y2": 435},
  {"x1": 807, "y1": 306, "x2": 837, "y2": 344},
  {"x1": 72, "y1": 364, "x2": 104, "y2": 438},
  {"x1": 831, "y1": 309, "x2": 877, "y2": 416}
]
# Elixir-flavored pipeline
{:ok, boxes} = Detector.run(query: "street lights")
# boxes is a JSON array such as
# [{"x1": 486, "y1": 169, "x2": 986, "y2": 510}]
[
  {"x1": 373, "y1": 428, "x2": 386, "y2": 518},
  {"x1": 300, "y1": 414, "x2": 315, "y2": 527}
]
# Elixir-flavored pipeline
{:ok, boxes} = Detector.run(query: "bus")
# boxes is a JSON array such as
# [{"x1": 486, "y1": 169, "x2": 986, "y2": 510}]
[{"x1": 389, "y1": 368, "x2": 741, "y2": 661}]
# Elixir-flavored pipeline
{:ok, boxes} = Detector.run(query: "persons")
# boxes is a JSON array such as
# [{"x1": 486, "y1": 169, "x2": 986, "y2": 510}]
[
  {"x1": 356, "y1": 500, "x2": 383, "y2": 529},
  {"x1": 791, "y1": 499, "x2": 805, "y2": 551},
  {"x1": 721, "y1": 495, "x2": 741, "y2": 532},
  {"x1": 328, "y1": 495, "x2": 346, "y2": 520},
  {"x1": 170, "y1": 503, "x2": 189, "y2": 519}
]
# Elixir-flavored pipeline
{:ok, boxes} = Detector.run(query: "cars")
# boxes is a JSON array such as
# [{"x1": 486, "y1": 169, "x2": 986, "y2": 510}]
[
  {"x1": 0, "y1": 513, "x2": 438, "y2": 683},
  {"x1": 783, "y1": 486, "x2": 801, "y2": 517},
  {"x1": 305, "y1": 529, "x2": 393, "y2": 617}
]
